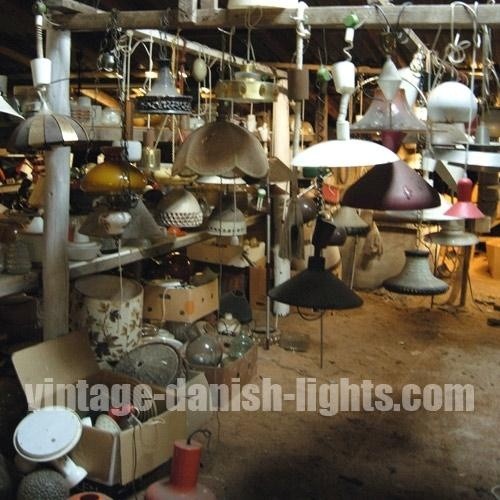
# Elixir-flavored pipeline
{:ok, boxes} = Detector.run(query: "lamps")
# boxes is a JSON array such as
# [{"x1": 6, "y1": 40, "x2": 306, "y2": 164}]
[
  {"x1": 72, "y1": 275, "x2": 147, "y2": 368},
  {"x1": 0, "y1": 2, "x2": 484, "y2": 319}
]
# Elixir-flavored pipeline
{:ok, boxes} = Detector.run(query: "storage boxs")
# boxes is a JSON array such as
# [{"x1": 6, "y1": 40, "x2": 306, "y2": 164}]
[
  {"x1": 184, "y1": 342, "x2": 257, "y2": 407},
  {"x1": 12, "y1": 329, "x2": 185, "y2": 486},
  {"x1": 167, "y1": 370, "x2": 215, "y2": 438}
]
